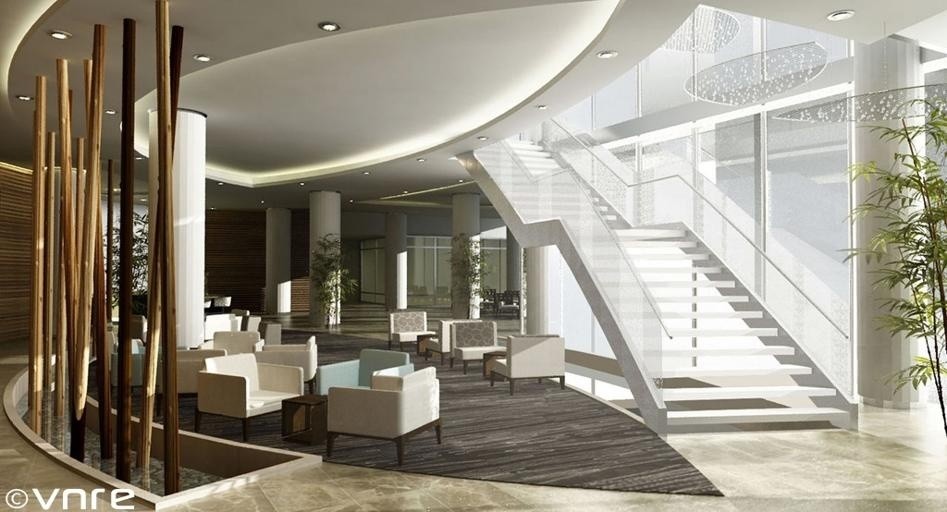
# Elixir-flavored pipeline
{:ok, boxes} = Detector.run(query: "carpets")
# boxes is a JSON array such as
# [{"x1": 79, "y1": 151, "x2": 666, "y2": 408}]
[{"x1": 85, "y1": 328, "x2": 723, "y2": 498}]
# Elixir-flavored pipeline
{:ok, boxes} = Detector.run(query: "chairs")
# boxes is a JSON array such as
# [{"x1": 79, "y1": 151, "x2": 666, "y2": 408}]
[
  {"x1": 385, "y1": 307, "x2": 567, "y2": 397},
  {"x1": 103, "y1": 293, "x2": 327, "y2": 468},
  {"x1": 482, "y1": 287, "x2": 519, "y2": 321},
  {"x1": 324, "y1": 364, "x2": 444, "y2": 466},
  {"x1": 316, "y1": 347, "x2": 415, "y2": 399}
]
